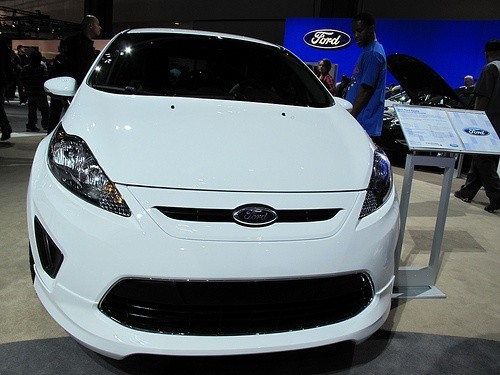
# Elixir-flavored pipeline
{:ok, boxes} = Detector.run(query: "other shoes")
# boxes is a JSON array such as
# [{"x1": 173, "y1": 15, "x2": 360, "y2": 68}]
[
  {"x1": 42, "y1": 126, "x2": 48, "y2": 133},
  {"x1": 26, "y1": 126, "x2": 40, "y2": 131},
  {"x1": 1, "y1": 126, "x2": 12, "y2": 140}
]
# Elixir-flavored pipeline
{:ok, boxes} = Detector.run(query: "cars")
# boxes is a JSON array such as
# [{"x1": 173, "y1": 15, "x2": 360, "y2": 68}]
[
  {"x1": 378, "y1": 53, "x2": 471, "y2": 173},
  {"x1": 24, "y1": 26, "x2": 402, "y2": 361}
]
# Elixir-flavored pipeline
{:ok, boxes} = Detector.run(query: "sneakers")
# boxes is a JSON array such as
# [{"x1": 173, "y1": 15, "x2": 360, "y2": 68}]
[
  {"x1": 484, "y1": 204, "x2": 500, "y2": 214},
  {"x1": 455, "y1": 191, "x2": 472, "y2": 203}
]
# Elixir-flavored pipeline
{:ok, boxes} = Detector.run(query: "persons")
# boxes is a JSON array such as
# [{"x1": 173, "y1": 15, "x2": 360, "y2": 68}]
[
  {"x1": 317, "y1": 59, "x2": 336, "y2": 95},
  {"x1": 0, "y1": 14, "x2": 102, "y2": 141},
  {"x1": 459, "y1": 75, "x2": 478, "y2": 109},
  {"x1": 455, "y1": 41, "x2": 500, "y2": 213},
  {"x1": 336, "y1": 76, "x2": 352, "y2": 97},
  {"x1": 345, "y1": 13, "x2": 388, "y2": 137}
]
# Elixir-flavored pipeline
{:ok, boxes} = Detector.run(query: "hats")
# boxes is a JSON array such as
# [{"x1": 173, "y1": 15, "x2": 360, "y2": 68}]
[
  {"x1": 464, "y1": 75, "x2": 473, "y2": 82},
  {"x1": 485, "y1": 39, "x2": 500, "y2": 52}
]
